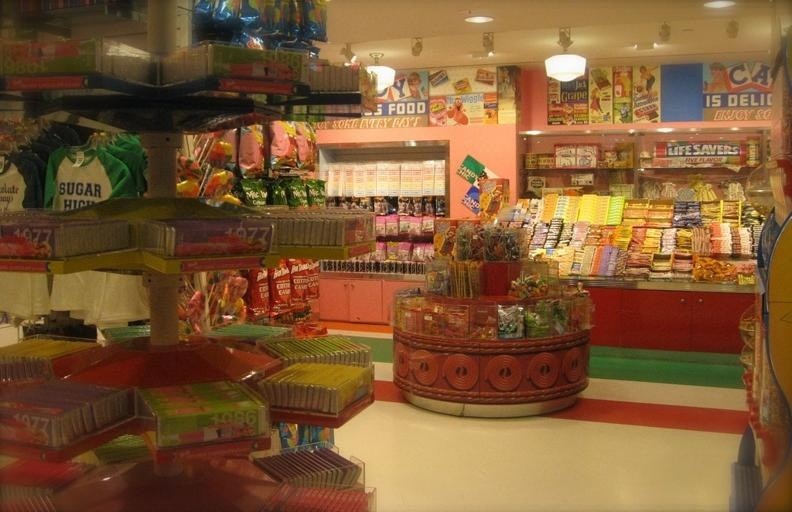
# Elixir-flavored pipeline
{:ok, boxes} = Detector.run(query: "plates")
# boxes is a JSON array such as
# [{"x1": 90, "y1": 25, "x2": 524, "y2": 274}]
[
  {"x1": 621, "y1": 290, "x2": 755, "y2": 353},
  {"x1": 318, "y1": 275, "x2": 382, "y2": 325},
  {"x1": 583, "y1": 286, "x2": 622, "y2": 348},
  {"x1": 517, "y1": 126, "x2": 770, "y2": 200},
  {"x1": 320, "y1": 152, "x2": 446, "y2": 275},
  {"x1": 382, "y1": 283, "x2": 425, "y2": 326},
  {"x1": 0, "y1": 1, "x2": 378, "y2": 512}
]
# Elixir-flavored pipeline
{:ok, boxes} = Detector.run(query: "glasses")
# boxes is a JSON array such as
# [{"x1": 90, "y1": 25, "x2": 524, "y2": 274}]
[
  {"x1": 484, "y1": 34, "x2": 496, "y2": 57},
  {"x1": 412, "y1": 37, "x2": 422, "y2": 56},
  {"x1": 659, "y1": 22, "x2": 671, "y2": 44},
  {"x1": 727, "y1": 19, "x2": 740, "y2": 39},
  {"x1": 558, "y1": 30, "x2": 574, "y2": 47},
  {"x1": 544, "y1": 48, "x2": 587, "y2": 82},
  {"x1": 365, "y1": 53, "x2": 395, "y2": 87},
  {"x1": 341, "y1": 43, "x2": 356, "y2": 60}
]
